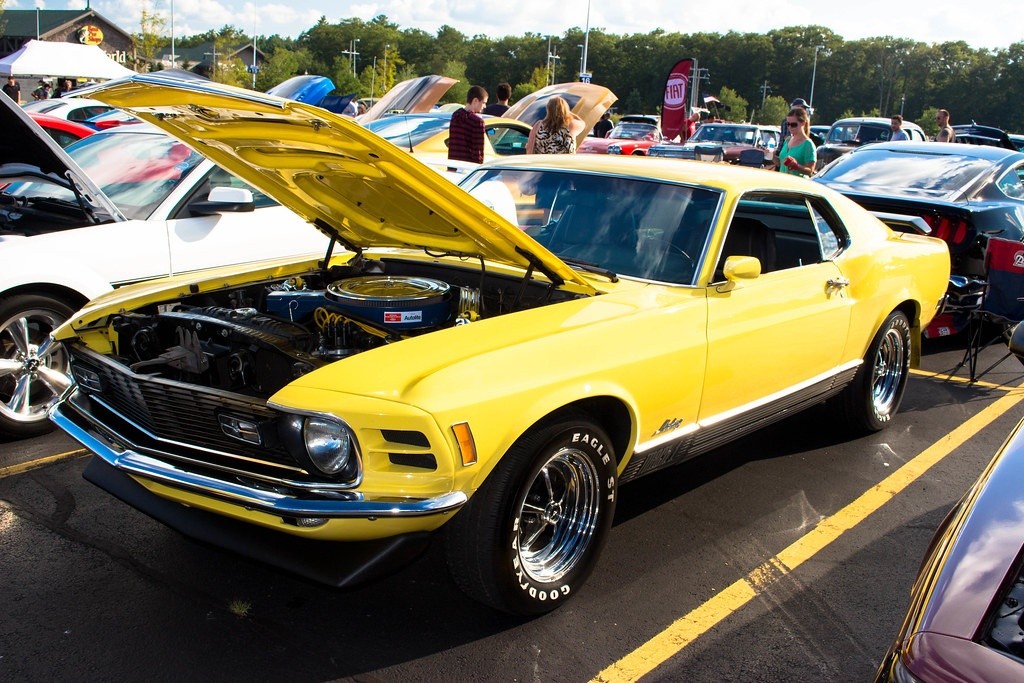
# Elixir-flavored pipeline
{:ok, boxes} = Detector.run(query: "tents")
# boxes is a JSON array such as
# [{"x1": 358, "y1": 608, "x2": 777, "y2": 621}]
[{"x1": 0, "y1": 39, "x2": 140, "y2": 81}]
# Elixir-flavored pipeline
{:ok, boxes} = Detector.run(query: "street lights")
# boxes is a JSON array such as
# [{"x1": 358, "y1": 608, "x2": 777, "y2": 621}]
[
  {"x1": 353, "y1": 39, "x2": 361, "y2": 78},
  {"x1": 546, "y1": 56, "x2": 561, "y2": 85},
  {"x1": 384, "y1": 45, "x2": 391, "y2": 84},
  {"x1": 810, "y1": 45, "x2": 826, "y2": 108},
  {"x1": 577, "y1": 45, "x2": 584, "y2": 78}
]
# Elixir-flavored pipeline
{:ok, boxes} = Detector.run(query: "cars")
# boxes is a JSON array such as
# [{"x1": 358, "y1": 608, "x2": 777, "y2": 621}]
[
  {"x1": 620, "y1": 114, "x2": 853, "y2": 171},
  {"x1": 807, "y1": 138, "x2": 1024, "y2": 322},
  {"x1": 576, "y1": 120, "x2": 664, "y2": 156},
  {"x1": 266, "y1": 75, "x2": 618, "y2": 223},
  {"x1": 48, "y1": 72, "x2": 952, "y2": 607},
  {"x1": 875, "y1": 318, "x2": 1023, "y2": 682},
  {"x1": 817, "y1": 118, "x2": 926, "y2": 172},
  {"x1": 0, "y1": 86, "x2": 514, "y2": 436},
  {"x1": 954, "y1": 125, "x2": 1024, "y2": 184}
]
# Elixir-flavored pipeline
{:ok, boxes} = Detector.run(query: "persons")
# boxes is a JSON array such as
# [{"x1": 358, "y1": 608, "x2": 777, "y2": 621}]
[
  {"x1": 679, "y1": 113, "x2": 700, "y2": 144},
  {"x1": 774, "y1": 98, "x2": 817, "y2": 180},
  {"x1": 526, "y1": 97, "x2": 577, "y2": 155},
  {"x1": 481, "y1": 83, "x2": 512, "y2": 117},
  {"x1": 934, "y1": 109, "x2": 956, "y2": 143},
  {"x1": 31, "y1": 80, "x2": 81, "y2": 101},
  {"x1": 593, "y1": 113, "x2": 614, "y2": 139},
  {"x1": 2, "y1": 76, "x2": 21, "y2": 106},
  {"x1": 447, "y1": 86, "x2": 488, "y2": 176},
  {"x1": 890, "y1": 115, "x2": 910, "y2": 142},
  {"x1": 762, "y1": 132, "x2": 776, "y2": 166},
  {"x1": 707, "y1": 114, "x2": 716, "y2": 123},
  {"x1": 342, "y1": 99, "x2": 366, "y2": 118}
]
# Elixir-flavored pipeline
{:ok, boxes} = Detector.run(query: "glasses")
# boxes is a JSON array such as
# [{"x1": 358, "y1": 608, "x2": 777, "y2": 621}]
[
  {"x1": 786, "y1": 122, "x2": 798, "y2": 127},
  {"x1": 934, "y1": 117, "x2": 940, "y2": 120}
]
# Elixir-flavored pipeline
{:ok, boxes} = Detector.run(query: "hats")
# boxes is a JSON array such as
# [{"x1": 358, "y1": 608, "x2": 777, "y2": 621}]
[{"x1": 792, "y1": 98, "x2": 811, "y2": 108}]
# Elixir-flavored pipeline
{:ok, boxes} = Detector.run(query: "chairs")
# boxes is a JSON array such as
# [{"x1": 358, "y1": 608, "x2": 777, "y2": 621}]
[
  {"x1": 551, "y1": 205, "x2": 770, "y2": 274},
  {"x1": 943, "y1": 238, "x2": 1023, "y2": 384}
]
